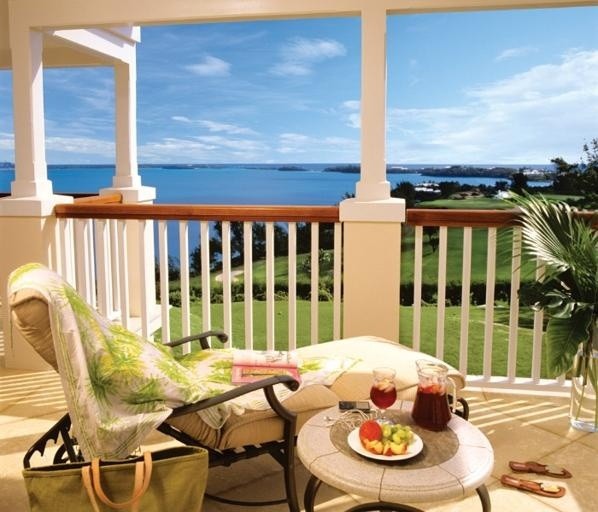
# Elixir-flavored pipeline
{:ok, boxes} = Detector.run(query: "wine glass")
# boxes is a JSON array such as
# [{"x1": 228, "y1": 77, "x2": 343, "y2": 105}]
[{"x1": 369, "y1": 366, "x2": 397, "y2": 427}]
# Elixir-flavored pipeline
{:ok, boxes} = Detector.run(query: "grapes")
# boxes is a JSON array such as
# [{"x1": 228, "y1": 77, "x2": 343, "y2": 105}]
[{"x1": 381, "y1": 423, "x2": 413, "y2": 443}]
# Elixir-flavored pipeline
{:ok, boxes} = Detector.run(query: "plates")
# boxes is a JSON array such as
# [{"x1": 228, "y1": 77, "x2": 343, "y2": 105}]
[{"x1": 347, "y1": 421, "x2": 424, "y2": 462}]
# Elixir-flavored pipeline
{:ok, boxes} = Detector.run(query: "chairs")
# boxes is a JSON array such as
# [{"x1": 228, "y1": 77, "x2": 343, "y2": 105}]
[{"x1": 6, "y1": 262, "x2": 469, "y2": 512}]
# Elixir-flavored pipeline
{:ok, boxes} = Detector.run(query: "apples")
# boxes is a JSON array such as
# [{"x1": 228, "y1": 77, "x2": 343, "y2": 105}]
[
  {"x1": 360, "y1": 420, "x2": 382, "y2": 445},
  {"x1": 364, "y1": 440, "x2": 409, "y2": 455}
]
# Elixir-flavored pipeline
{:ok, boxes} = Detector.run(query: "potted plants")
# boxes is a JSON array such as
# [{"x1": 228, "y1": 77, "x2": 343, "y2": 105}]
[{"x1": 491, "y1": 188, "x2": 598, "y2": 433}]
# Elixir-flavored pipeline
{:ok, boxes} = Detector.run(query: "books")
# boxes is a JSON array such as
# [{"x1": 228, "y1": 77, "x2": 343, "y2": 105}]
[{"x1": 231, "y1": 363, "x2": 303, "y2": 384}]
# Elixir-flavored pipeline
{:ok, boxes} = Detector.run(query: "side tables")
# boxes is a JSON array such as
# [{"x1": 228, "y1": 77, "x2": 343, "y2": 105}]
[{"x1": 295, "y1": 400, "x2": 495, "y2": 512}]
